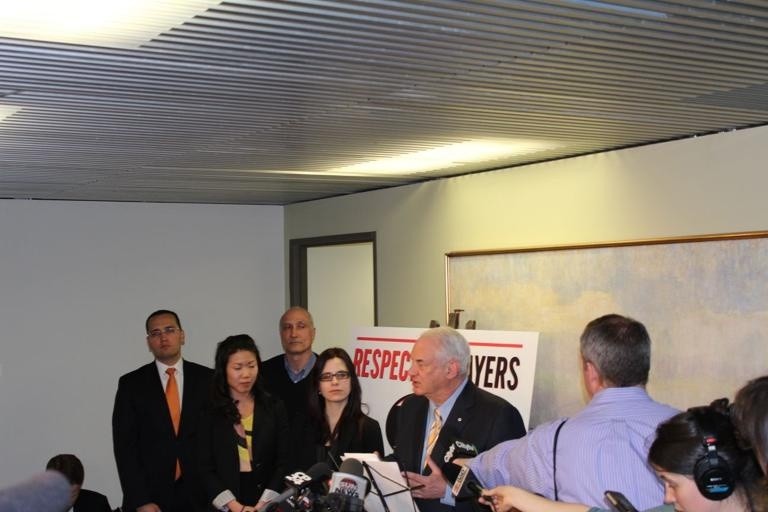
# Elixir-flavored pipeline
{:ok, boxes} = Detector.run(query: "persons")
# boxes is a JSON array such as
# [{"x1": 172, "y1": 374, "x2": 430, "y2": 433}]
[
  {"x1": 112, "y1": 309, "x2": 218, "y2": 512},
  {"x1": 178, "y1": 333, "x2": 295, "y2": 512},
  {"x1": 478, "y1": 398, "x2": 758, "y2": 512},
  {"x1": 375, "y1": 324, "x2": 527, "y2": 511},
  {"x1": 44, "y1": 453, "x2": 113, "y2": 512},
  {"x1": 452, "y1": 313, "x2": 687, "y2": 512},
  {"x1": 255, "y1": 305, "x2": 320, "y2": 415},
  {"x1": 295, "y1": 347, "x2": 385, "y2": 471},
  {"x1": 729, "y1": 375, "x2": 768, "y2": 512}
]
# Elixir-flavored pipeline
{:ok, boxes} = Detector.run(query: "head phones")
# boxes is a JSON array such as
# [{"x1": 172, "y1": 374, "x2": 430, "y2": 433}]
[{"x1": 694, "y1": 431, "x2": 735, "y2": 501}]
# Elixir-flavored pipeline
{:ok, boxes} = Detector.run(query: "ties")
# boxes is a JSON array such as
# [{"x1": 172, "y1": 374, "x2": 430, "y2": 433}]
[
  {"x1": 424, "y1": 408, "x2": 442, "y2": 469},
  {"x1": 165, "y1": 368, "x2": 181, "y2": 480}
]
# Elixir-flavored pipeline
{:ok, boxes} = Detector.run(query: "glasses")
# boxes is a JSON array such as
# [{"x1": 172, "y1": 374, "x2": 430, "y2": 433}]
[{"x1": 320, "y1": 372, "x2": 349, "y2": 381}]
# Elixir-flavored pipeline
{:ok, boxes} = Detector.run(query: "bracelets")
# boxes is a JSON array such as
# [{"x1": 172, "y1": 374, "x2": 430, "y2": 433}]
[{"x1": 240, "y1": 504, "x2": 245, "y2": 512}]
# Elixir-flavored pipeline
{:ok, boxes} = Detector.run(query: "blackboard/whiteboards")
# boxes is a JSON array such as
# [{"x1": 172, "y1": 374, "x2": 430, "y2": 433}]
[{"x1": 444, "y1": 230, "x2": 768, "y2": 429}]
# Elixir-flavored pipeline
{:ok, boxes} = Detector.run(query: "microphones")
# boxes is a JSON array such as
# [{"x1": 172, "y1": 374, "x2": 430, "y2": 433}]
[
  {"x1": 317, "y1": 459, "x2": 368, "y2": 512},
  {"x1": 271, "y1": 463, "x2": 332, "y2": 505},
  {"x1": 440, "y1": 429, "x2": 478, "y2": 463},
  {"x1": 442, "y1": 462, "x2": 492, "y2": 501}
]
